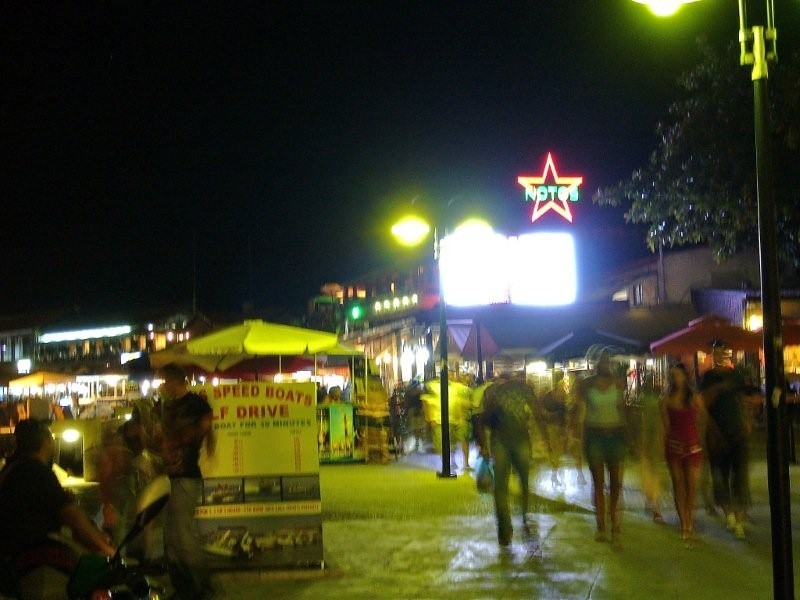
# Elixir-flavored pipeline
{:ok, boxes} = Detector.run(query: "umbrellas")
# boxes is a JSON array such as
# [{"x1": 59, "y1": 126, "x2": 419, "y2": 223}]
[
  {"x1": 6, "y1": 370, "x2": 77, "y2": 396},
  {"x1": 649, "y1": 311, "x2": 762, "y2": 352},
  {"x1": 542, "y1": 325, "x2": 643, "y2": 368},
  {"x1": 123, "y1": 318, "x2": 340, "y2": 383}
]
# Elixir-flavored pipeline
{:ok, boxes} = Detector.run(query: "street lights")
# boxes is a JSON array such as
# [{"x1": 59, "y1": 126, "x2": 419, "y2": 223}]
[
  {"x1": 637, "y1": 0, "x2": 797, "y2": 600},
  {"x1": 390, "y1": 192, "x2": 457, "y2": 480}
]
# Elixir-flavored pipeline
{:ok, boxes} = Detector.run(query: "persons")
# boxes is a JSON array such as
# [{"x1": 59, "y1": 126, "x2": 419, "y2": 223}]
[
  {"x1": 94, "y1": 362, "x2": 213, "y2": 576},
  {"x1": 385, "y1": 368, "x2": 493, "y2": 470},
  {"x1": 0, "y1": 419, "x2": 117, "y2": 559},
  {"x1": 536, "y1": 343, "x2": 635, "y2": 545},
  {"x1": 657, "y1": 361, "x2": 710, "y2": 542},
  {"x1": 477, "y1": 353, "x2": 554, "y2": 547},
  {"x1": 659, "y1": 336, "x2": 763, "y2": 542},
  {"x1": 316, "y1": 381, "x2": 344, "y2": 404}
]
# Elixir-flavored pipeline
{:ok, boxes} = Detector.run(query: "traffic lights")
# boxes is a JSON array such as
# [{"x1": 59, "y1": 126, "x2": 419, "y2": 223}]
[{"x1": 350, "y1": 303, "x2": 363, "y2": 321}]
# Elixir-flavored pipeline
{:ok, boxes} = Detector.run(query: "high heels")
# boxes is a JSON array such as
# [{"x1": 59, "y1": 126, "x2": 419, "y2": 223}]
[
  {"x1": 576, "y1": 473, "x2": 588, "y2": 485},
  {"x1": 550, "y1": 475, "x2": 562, "y2": 487}
]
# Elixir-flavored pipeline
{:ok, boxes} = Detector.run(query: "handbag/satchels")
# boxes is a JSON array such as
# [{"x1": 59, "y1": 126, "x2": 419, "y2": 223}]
[{"x1": 476, "y1": 459, "x2": 494, "y2": 493}]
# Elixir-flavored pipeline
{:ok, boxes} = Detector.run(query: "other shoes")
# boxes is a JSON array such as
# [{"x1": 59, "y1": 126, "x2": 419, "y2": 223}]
[
  {"x1": 525, "y1": 527, "x2": 538, "y2": 537},
  {"x1": 680, "y1": 527, "x2": 694, "y2": 550},
  {"x1": 726, "y1": 512, "x2": 736, "y2": 530},
  {"x1": 597, "y1": 528, "x2": 608, "y2": 542},
  {"x1": 611, "y1": 522, "x2": 621, "y2": 535},
  {"x1": 497, "y1": 538, "x2": 512, "y2": 545},
  {"x1": 736, "y1": 520, "x2": 747, "y2": 540}
]
